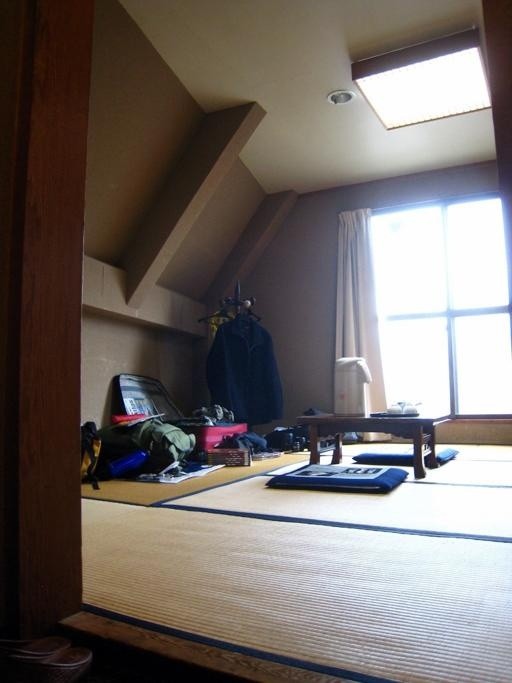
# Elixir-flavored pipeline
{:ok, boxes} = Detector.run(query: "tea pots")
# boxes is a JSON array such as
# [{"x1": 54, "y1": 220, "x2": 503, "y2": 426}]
[
  {"x1": 384, "y1": 404, "x2": 402, "y2": 413},
  {"x1": 402, "y1": 403, "x2": 424, "y2": 413}
]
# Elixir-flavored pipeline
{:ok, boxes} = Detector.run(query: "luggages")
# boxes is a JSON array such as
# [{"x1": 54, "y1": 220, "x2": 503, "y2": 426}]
[{"x1": 117, "y1": 372, "x2": 248, "y2": 461}]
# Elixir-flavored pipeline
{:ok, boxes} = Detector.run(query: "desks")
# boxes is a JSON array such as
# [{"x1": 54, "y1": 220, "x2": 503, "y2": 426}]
[{"x1": 297, "y1": 411, "x2": 454, "y2": 479}]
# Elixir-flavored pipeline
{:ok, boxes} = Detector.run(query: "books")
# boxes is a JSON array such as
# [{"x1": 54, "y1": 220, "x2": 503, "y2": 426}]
[{"x1": 207, "y1": 447, "x2": 251, "y2": 467}]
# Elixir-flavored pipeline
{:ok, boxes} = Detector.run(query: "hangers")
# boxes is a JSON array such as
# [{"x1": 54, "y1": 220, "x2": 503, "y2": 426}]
[{"x1": 197, "y1": 297, "x2": 262, "y2": 326}]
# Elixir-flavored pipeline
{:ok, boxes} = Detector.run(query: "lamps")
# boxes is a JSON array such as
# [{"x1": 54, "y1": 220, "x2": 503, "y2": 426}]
[{"x1": 348, "y1": 28, "x2": 493, "y2": 131}]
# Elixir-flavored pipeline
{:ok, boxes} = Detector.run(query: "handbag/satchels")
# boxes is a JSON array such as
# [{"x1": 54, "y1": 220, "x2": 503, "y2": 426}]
[
  {"x1": 81, "y1": 422, "x2": 103, "y2": 490},
  {"x1": 98, "y1": 418, "x2": 197, "y2": 478}
]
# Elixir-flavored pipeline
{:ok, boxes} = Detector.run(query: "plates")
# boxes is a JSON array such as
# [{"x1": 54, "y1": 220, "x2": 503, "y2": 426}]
[{"x1": 371, "y1": 411, "x2": 423, "y2": 420}]
